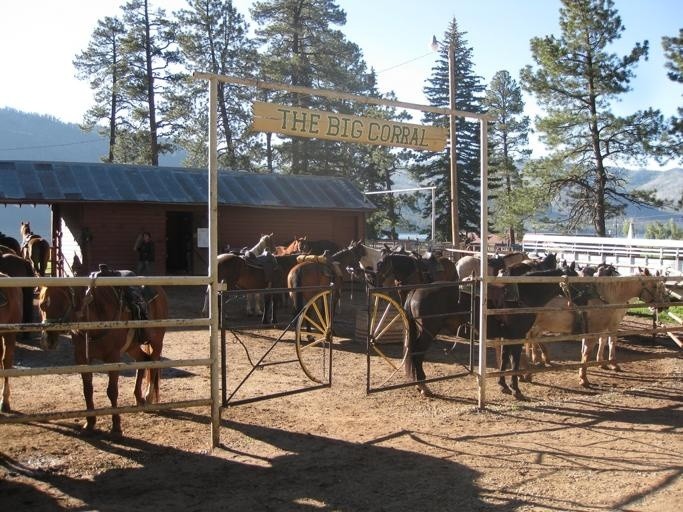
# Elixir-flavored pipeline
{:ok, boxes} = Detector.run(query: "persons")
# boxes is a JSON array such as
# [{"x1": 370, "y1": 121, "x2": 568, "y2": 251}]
[
  {"x1": 465, "y1": 232, "x2": 479, "y2": 251},
  {"x1": 134, "y1": 232, "x2": 156, "y2": 275}
]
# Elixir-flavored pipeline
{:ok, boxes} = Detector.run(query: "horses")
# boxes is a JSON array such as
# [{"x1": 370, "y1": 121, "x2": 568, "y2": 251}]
[
  {"x1": 39, "y1": 285, "x2": 170, "y2": 440},
  {"x1": 347, "y1": 239, "x2": 670, "y2": 399},
  {"x1": 201, "y1": 232, "x2": 361, "y2": 342},
  {"x1": 0, "y1": 221, "x2": 49, "y2": 413}
]
